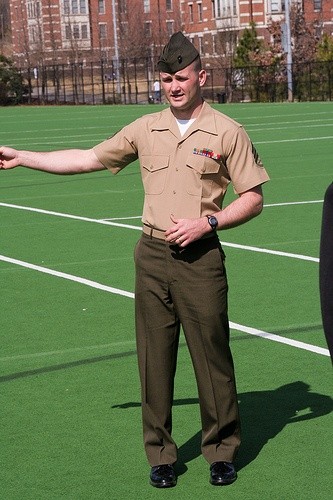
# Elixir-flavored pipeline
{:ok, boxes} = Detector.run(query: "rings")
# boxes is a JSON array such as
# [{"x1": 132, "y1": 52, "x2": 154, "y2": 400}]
[{"x1": 179, "y1": 238, "x2": 182, "y2": 243}]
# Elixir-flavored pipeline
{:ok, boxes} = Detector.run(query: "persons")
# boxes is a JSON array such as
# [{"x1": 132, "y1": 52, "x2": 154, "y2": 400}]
[
  {"x1": 0, "y1": 30, "x2": 270, "y2": 487},
  {"x1": 153, "y1": 77, "x2": 163, "y2": 101}
]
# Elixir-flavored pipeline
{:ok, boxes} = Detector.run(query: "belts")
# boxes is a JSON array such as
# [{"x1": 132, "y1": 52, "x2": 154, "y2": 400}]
[{"x1": 142, "y1": 225, "x2": 216, "y2": 242}]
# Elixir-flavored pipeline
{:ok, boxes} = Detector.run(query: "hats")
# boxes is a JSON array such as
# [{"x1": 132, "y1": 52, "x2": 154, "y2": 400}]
[{"x1": 157, "y1": 30, "x2": 199, "y2": 74}]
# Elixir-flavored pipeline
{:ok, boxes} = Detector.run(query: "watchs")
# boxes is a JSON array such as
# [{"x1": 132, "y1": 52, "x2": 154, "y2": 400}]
[{"x1": 207, "y1": 215, "x2": 219, "y2": 231}]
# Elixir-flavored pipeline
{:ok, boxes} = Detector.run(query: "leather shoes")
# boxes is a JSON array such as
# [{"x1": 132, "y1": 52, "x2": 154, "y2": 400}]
[
  {"x1": 209, "y1": 461, "x2": 237, "y2": 486},
  {"x1": 148, "y1": 464, "x2": 177, "y2": 488}
]
